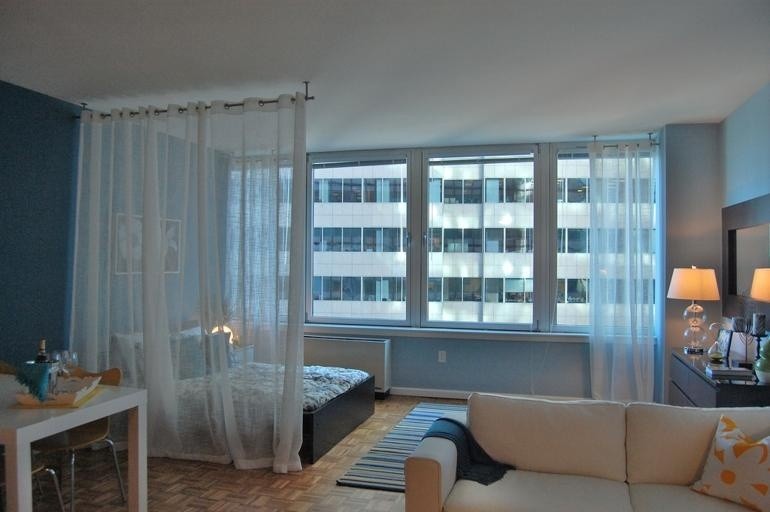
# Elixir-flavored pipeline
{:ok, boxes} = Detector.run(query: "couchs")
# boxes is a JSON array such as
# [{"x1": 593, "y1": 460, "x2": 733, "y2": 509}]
[{"x1": 405, "y1": 392, "x2": 770, "y2": 512}]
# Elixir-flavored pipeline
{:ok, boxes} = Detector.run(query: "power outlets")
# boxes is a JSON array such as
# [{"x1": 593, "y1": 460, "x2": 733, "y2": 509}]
[{"x1": 438, "y1": 350, "x2": 447, "y2": 363}]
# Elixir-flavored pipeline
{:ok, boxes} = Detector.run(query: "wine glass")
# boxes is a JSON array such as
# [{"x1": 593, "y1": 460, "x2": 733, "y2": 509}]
[
  {"x1": 53, "y1": 350, "x2": 71, "y2": 396},
  {"x1": 59, "y1": 347, "x2": 80, "y2": 393}
]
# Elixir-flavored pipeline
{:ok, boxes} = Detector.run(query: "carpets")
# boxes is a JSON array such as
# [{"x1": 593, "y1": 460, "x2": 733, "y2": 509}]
[{"x1": 335, "y1": 401, "x2": 468, "y2": 494}]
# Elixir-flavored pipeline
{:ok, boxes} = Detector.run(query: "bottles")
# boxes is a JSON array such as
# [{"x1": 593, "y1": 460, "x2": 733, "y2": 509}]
[{"x1": 35, "y1": 337, "x2": 49, "y2": 363}]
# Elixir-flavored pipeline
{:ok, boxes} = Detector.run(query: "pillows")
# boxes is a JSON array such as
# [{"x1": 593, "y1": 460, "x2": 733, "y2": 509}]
[{"x1": 690, "y1": 411, "x2": 770, "y2": 512}]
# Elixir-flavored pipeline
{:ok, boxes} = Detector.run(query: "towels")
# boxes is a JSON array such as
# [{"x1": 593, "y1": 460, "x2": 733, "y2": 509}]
[{"x1": 14, "y1": 363, "x2": 50, "y2": 403}]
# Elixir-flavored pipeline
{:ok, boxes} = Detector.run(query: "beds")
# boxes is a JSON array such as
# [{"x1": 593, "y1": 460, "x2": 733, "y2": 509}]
[{"x1": 101, "y1": 330, "x2": 376, "y2": 465}]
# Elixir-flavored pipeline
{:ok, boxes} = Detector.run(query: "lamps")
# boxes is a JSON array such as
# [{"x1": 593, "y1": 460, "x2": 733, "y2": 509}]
[
  {"x1": 210, "y1": 326, "x2": 234, "y2": 347},
  {"x1": 750, "y1": 268, "x2": 770, "y2": 388},
  {"x1": 666, "y1": 264, "x2": 721, "y2": 355}
]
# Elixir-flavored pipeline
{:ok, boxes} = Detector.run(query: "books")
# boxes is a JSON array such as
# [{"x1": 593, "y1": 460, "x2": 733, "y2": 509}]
[{"x1": 706, "y1": 365, "x2": 752, "y2": 377}]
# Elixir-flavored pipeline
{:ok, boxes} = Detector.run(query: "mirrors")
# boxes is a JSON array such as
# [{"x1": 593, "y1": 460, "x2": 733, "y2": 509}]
[{"x1": 721, "y1": 193, "x2": 770, "y2": 334}]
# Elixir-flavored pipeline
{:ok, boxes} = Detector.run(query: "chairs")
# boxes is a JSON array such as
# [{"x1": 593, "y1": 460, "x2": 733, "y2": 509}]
[
  {"x1": 0, "y1": 441, "x2": 66, "y2": 512},
  {"x1": 45, "y1": 367, "x2": 127, "y2": 512}
]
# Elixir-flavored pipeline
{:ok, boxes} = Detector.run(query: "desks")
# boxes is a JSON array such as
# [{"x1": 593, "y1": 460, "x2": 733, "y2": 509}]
[{"x1": 0, "y1": 383, "x2": 149, "y2": 512}]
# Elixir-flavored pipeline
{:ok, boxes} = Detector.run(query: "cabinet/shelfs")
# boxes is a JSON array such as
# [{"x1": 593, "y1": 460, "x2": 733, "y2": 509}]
[{"x1": 667, "y1": 346, "x2": 770, "y2": 408}]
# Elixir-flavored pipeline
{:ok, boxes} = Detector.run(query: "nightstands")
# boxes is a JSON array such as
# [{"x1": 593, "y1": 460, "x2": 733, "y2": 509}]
[{"x1": 233, "y1": 344, "x2": 254, "y2": 363}]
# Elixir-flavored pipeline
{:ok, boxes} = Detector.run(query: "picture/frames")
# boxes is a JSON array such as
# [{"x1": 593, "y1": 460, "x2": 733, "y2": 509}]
[{"x1": 112, "y1": 211, "x2": 182, "y2": 277}]
